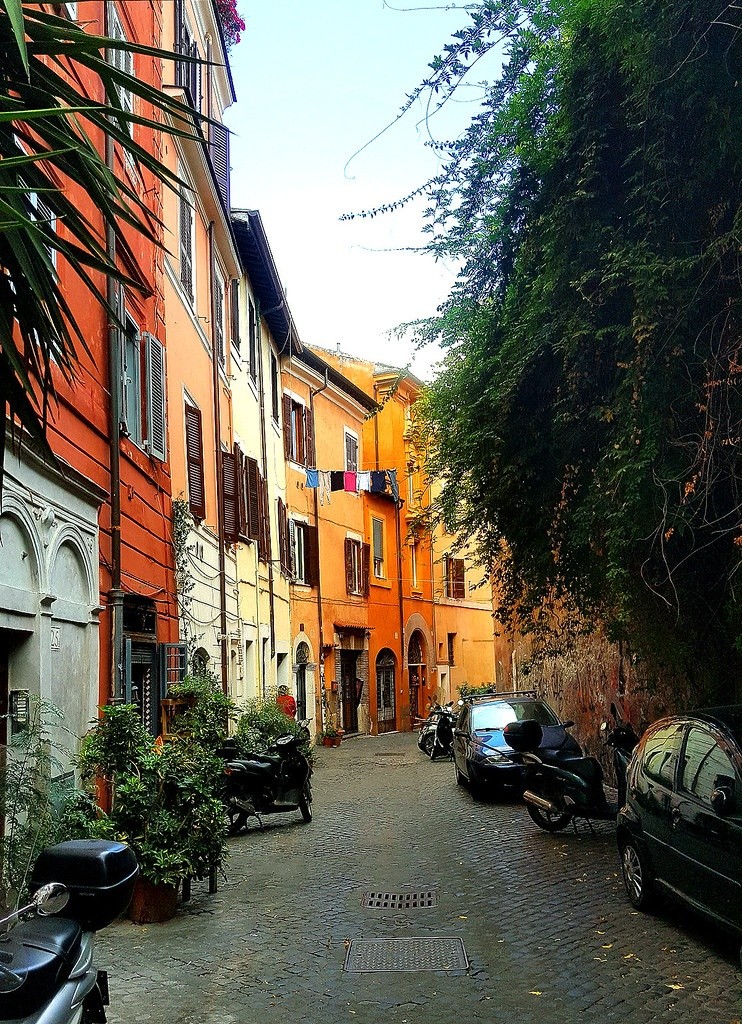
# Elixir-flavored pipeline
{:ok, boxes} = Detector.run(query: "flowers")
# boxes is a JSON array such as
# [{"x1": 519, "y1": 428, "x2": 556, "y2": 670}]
[{"x1": 216, "y1": 0, "x2": 246, "y2": 57}]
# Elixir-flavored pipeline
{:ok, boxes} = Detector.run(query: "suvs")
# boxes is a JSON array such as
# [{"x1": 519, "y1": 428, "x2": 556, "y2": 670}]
[{"x1": 617, "y1": 706, "x2": 742, "y2": 938}]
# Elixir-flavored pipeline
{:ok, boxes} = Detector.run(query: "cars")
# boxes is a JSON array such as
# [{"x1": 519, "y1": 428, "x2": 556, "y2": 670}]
[{"x1": 452, "y1": 690, "x2": 574, "y2": 802}]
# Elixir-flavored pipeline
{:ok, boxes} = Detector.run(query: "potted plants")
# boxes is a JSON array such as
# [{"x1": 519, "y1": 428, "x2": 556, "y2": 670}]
[
  {"x1": 319, "y1": 725, "x2": 343, "y2": 747},
  {"x1": 64, "y1": 745, "x2": 229, "y2": 925}
]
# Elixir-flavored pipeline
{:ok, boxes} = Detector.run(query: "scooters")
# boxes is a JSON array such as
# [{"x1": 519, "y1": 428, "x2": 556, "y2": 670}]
[
  {"x1": 417, "y1": 695, "x2": 463, "y2": 761},
  {"x1": 0, "y1": 838, "x2": 139, "y2": 1023}
]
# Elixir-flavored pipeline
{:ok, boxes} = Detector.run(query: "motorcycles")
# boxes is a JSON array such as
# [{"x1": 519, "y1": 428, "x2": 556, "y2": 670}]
[
  {"x1": 503, "y1": 703, "x2": 639, "y2": 836},
  {"x1": 216, "y1": 718, "x2": 313, "y2": 836}
]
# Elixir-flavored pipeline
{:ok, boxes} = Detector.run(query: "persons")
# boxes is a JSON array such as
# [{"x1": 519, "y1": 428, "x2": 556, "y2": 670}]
[{"x1": 275, "y1": 684, "x2": 298, "y2": 721}]
